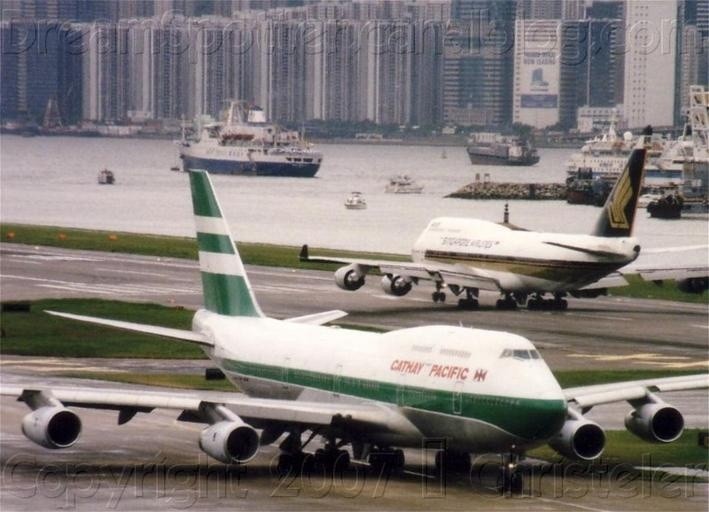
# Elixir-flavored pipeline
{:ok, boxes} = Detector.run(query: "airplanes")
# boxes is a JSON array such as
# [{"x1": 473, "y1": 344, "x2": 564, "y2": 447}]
[
  {"x1": 297, "y1": 123, "x2": 654, "y2": 313},
  {"x1": 16, "y1": 166, "x2": 709, "y2": 496}
]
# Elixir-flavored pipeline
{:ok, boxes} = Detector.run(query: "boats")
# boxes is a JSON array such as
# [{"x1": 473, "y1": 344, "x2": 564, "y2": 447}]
[
  {"x1": 178, "y1": 100, "x2": 324, "y2": 177},
  {"x1": 467, "y1": 138, "x2": 540, "y2": 166},
  {"x1": 98, "y1": 170, "x2": 113, "y2": 184},
  {"x1": 344, "y1": 175, "x2": 424, "y2": 210},
  {"x1": 566, "y1": 84, "x2": 708, "y2": 220}
]
